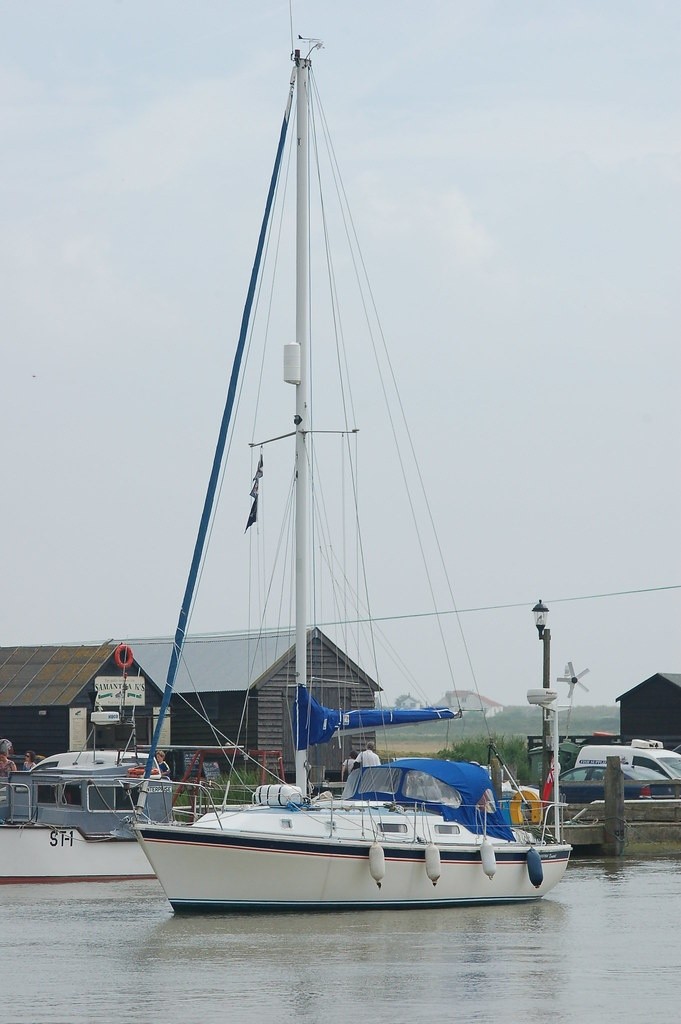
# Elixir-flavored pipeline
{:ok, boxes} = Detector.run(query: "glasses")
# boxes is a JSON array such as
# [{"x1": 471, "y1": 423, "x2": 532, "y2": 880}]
[{"x1": 25, "y1": 754, "x2": 29, "y2": 758}]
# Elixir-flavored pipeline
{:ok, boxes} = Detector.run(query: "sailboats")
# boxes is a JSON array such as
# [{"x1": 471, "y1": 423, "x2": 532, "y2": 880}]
[{"x1": 126, "y1": 34, "x2": 573, "y2": 913}]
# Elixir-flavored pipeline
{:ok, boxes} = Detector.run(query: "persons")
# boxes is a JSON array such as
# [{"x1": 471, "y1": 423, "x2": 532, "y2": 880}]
[
  {"x1": 342, "y1": 751, "x2": 357, "y2": 776},
  {"x1": 156, "y1": 750, "x2": 171, "y2": 780},
  {"x1": 355, "y1": 741, "x2": 381, "y2": 768},
  {"x1": 0, "y1": 745, "x2": 17, "y2": 777},
  {"x1": 23, "y1": 751, "x2": 36, "y2": 771}
]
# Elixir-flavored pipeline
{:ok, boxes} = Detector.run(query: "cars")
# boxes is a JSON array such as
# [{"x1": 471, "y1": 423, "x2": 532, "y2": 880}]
[{"x1": 559, "y1": 763, "x2": 681, "y2": 804}]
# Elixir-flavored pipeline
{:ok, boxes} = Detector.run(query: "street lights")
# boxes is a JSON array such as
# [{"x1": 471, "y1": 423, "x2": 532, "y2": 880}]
[{"x1": 533, "y1": 598, "x2": 549, "y2": 813}]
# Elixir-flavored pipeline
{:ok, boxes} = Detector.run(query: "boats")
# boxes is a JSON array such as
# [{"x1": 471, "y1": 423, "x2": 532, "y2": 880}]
[{"x1": 0, "y1": 711, "x2": 190, "y2": 886}]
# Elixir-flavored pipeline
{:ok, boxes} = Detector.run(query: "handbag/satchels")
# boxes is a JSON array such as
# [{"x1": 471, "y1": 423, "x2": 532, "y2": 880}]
[
  {"x1": 351, "y1": 762, "x2": 360, "y2": 772},
  {"x1": 343, "y1": 770, "x2": 348, "y2": 782}
]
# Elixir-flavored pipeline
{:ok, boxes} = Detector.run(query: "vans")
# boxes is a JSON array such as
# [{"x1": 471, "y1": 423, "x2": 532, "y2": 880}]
[{"x1": 574, "y1": 738, "x2": 681, "y2": 780}]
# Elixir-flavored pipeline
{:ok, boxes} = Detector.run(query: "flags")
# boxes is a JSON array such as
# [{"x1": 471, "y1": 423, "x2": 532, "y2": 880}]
[{"x1": 542, "y1": 761, "x2": 554, "y2": 806}]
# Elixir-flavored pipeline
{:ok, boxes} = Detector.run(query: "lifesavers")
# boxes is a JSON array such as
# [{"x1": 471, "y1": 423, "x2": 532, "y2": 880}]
[
  {"x1": 114, "y1": 644, "x2": 133, "y2": 670},
  {"x1": 127, "y1": 766, "x2": 162, "y2": 782},
  {"x1": 510, "y1": 790, "x2": 543, "y2": 823}
]
